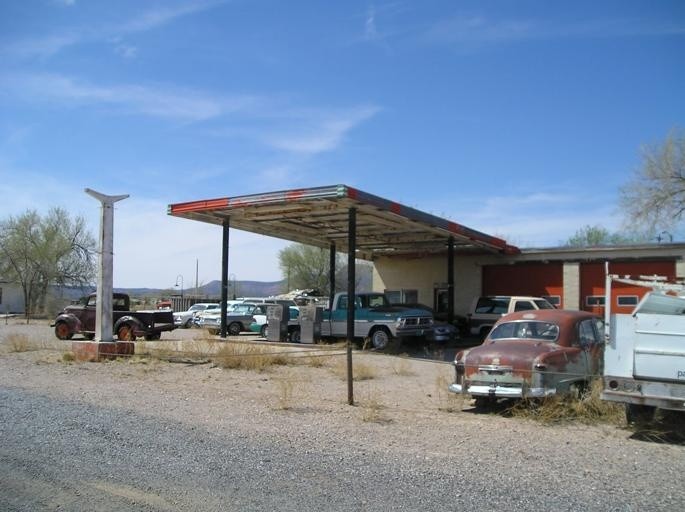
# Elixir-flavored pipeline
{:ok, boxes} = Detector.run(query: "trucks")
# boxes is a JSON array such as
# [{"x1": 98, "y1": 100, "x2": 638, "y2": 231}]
[{"x1": 51, "y1": 290, "x2": 177, "y2": 344}]
